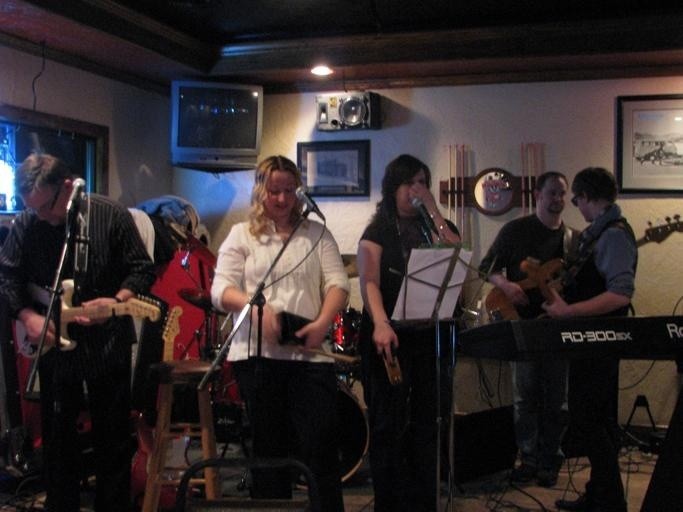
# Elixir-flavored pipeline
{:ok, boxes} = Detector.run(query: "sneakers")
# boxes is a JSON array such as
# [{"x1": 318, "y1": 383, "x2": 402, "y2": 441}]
[{"x1": 512, "y1": 462, "x2": 558, "y2": 486}]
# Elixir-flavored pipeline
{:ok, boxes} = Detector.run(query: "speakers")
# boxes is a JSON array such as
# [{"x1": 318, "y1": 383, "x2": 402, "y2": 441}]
[{"x1": 314, "y1": 91, "x2": 383, "y2": 132}]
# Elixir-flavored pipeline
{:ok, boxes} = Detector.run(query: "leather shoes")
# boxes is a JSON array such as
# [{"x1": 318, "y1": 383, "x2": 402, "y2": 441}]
[{"x1": 555, "y1": 494, "x2": 628, "y2": 512}]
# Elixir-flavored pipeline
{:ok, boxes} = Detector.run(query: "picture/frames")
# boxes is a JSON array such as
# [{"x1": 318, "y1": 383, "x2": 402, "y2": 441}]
[
  {"x1": 616, "y1": 94, "x2": 683, "y2": 194},
  {"x1": 297, "y1": 139, "x2": 370, "y2": 197}
]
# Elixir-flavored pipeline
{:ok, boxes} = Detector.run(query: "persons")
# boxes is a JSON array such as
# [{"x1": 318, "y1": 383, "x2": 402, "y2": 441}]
[
  {"x1": 354, "y1": 153, "x2": 463, "y2": 512},
  {"x1": 1, "y1": 152, "x2": 157, "y2": 511},
  {"x1": 206, "y1": 154, "x2": 351, "y2": 510},
  {"x1": 136, "y1": 192, "x2": 255, "y2": 438},
  {"x1": 540, "y1": 165, "x2": 638, "y2": 511},
  {"x1": 477, "y1": 171, "x2": 581, "y2": 488}
]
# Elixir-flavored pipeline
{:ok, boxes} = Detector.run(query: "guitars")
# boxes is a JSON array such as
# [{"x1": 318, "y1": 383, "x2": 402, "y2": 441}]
[
  {"x1": 484, "y1": 214, "x2": 683, "y2": 324},
  {"x1": 12, "y1": 280, "x2": 162, "y2": 358},
  {"x1": 133, "y1": 306, "x2": 191, "y2": 512}
]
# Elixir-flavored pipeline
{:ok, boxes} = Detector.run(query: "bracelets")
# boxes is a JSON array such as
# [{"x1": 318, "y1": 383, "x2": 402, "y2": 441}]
[
  {"x1": 427, "y1": 207, "x2": 439, "y2": 219},
  {"x1": 438, "y1": 221, "x2": 448, "y2": 235},
  {"x1": 373, "y1": 316, "x2": 390, "y2": 329},
  {"x1": 113, "y1": 296, "x2": 121, "y2": 303}
]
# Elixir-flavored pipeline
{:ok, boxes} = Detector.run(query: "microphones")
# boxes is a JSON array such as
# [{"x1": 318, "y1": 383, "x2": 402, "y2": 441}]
[
  {"x1": 294, "y1": 186, "x2": 326, "y2": 221},
  {"x1": 65, "y1": 176, "x2": 86, "y2": 217},
  {"x1": 411, "y1": 197, "x2": 439, "y2": 237}
]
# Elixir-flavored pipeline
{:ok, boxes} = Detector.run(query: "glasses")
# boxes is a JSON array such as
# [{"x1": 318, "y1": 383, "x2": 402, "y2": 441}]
[{"x1": 24, "y1": 184, "x2": 62, "y2": 215}]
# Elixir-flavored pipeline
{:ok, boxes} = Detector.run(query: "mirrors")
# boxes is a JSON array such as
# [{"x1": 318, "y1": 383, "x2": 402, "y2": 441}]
[{"x1": 471, "y1": 167, "x2": 518, "y2": 216}]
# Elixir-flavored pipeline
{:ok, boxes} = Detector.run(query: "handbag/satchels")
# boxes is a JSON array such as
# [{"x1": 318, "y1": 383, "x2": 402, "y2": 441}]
[{"x1": 273, "y1": 310, "x2": 313, "y2": 346}]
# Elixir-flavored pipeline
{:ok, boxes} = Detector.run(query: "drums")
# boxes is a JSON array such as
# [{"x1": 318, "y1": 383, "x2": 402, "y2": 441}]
[
  {"x1": 296, "y1": 380, "x2": 370, "y2": 491},
  {"x1": 331, "y1": 307, "x2": 363, "y2": 355}
]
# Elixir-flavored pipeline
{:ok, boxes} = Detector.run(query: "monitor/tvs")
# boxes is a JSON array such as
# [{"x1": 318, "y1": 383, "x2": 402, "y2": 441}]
[
  {"x1": 0, "y1": 115, "x2": 97, "y2": 222},
  {"x1": 168, "y1": 80, "x2": 264, "y2": 169}
]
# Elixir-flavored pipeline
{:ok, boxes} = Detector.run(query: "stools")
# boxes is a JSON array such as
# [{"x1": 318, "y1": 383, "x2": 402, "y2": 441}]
[{"x1": 142, "y1": 361, "x2": 221, "y2": 512}]
